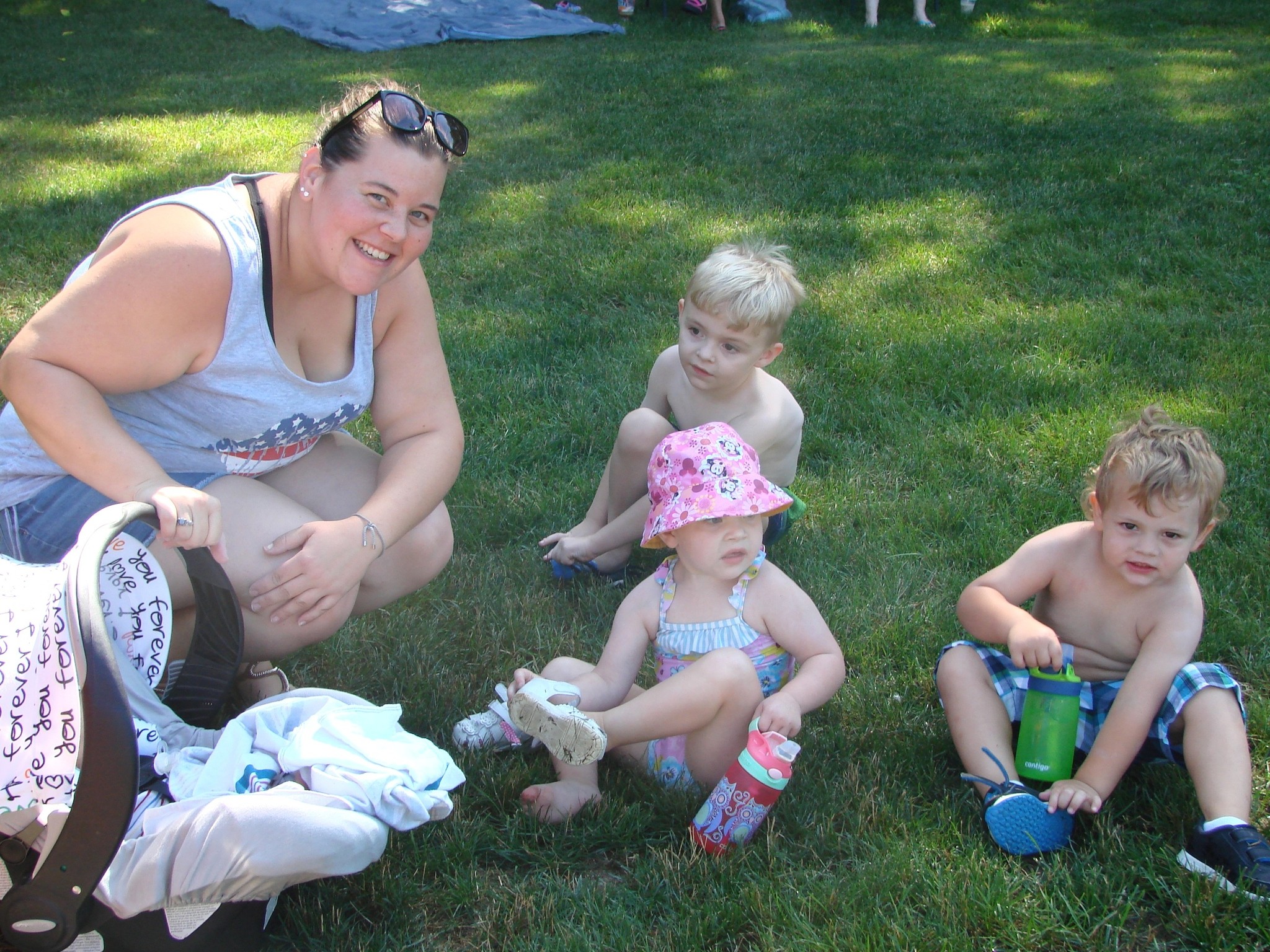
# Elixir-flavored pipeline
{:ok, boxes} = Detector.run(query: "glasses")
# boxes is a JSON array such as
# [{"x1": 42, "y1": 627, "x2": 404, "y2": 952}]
[{"x1": 317, "y1": 89, "x2": 469, "y2": 157}]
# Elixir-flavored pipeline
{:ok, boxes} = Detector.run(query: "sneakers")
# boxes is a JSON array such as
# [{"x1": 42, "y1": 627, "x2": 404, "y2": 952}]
[
  {"x1": 960, "y1": 743, "x2": 1076, "y2": 854},
  {"x1": 1176, "y1": 815, "x2": 1270, "y2": 903}
]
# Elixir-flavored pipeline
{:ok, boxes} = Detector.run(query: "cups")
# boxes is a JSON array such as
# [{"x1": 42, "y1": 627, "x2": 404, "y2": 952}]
[
  {"x1": 689, "y1": 716, "x2": 802, "y2": 860},
  {"x1": 1012, "y1": 642, "x2": 1082, "y2": 783}
]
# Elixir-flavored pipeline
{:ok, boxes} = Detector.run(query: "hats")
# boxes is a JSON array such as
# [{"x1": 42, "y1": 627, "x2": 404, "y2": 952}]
[{"x1": 639, "y1": 421, "x2": 793, "y2": 550}]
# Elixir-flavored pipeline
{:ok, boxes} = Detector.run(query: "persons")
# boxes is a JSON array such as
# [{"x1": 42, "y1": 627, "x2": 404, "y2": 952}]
[
  {"x1": 535, "y1": 241, "x2": 813, "y2": 591},
  {"x1": 934, "y1": 410, "x2": 1269, "y2": 905},
  {"x1": 1, "y1": 69, "x2": 468, "y2": 722},
  {"x1": 504, "y1": 425, "x2": 848, "y2": 826}
]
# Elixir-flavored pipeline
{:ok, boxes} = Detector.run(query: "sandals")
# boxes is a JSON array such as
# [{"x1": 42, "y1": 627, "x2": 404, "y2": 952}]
[
  {"x1": 452, "y1": 682, "x2": 533, "y2": 756},
  {"x1": 509, "y1": 678, "x2": 607, "y2": 767},
  {"x1": 551, "y1": 553, "x2": 634, "y2": 581}
]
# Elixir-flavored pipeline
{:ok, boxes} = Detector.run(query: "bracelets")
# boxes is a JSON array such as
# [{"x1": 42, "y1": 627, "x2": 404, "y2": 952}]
[{"x1": 350, "y1": 512, "x2": 389, "y2": 561}]
